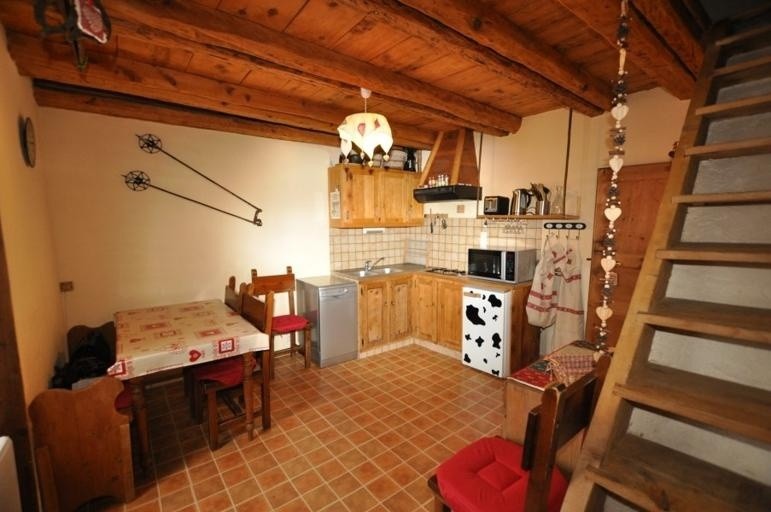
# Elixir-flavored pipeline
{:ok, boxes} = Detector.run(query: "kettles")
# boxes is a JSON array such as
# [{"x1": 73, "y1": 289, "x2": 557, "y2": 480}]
[{"x1": 510, "y1": 188, "x2": 531, "y2": 215}]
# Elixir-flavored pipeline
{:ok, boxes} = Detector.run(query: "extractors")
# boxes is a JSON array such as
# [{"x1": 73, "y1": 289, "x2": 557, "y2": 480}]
[{"x1": 413, "y1": 186, "x2": 483, "y2": 204}]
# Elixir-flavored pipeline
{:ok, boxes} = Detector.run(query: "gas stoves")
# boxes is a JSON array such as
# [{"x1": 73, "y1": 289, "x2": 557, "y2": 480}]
[{"x1": 425, "y1": 267, "x2": 465, "y2": 277}]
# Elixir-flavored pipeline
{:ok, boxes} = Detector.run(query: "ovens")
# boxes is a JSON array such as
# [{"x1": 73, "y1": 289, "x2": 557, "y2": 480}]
[{"x1": 296, "y1": 275, "x2": 359, "y2": 369}]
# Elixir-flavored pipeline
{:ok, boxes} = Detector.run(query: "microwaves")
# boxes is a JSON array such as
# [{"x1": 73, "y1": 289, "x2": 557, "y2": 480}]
[{"x1": 466, "y1": 247, "x2": 538, "y2": 285}]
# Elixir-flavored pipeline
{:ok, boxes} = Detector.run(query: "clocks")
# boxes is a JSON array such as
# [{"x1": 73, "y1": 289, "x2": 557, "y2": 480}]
[{"x1": 24, "y1": 118, "x2": 36, "y2": 167}]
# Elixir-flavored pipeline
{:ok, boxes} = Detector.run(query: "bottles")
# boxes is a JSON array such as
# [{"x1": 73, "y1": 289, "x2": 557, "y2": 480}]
[
  {"x1": 428, "y1": 174, "x2": 449, "y2": 188},
  {"x1": 550, "y1": 185, "x2": 563, "y2": 215}
]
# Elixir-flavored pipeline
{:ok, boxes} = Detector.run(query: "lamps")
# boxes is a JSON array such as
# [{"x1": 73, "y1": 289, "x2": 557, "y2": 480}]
[{"x1": 337, "y1": 88, "x2": 394, "y2": 170}]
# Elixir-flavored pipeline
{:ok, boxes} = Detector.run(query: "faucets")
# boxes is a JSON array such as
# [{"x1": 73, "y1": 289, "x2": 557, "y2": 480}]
[{"x1": 366, "y1": 258, "x2": 383, "y2": 271}]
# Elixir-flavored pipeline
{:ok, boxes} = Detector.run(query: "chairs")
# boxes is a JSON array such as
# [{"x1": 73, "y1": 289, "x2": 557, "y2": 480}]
[
  {"x1": 428, "y1": 354, "x2": 599, "y2": 511},
  {"x1": 182, "y1": 276, "x2": 246, "y2": 397},
  {"x1": 195, "y1": 285, "x2": 274, "y2": 450},
  {"x1": 250, "y1": 266, "x2": 315, "y2": 379}
]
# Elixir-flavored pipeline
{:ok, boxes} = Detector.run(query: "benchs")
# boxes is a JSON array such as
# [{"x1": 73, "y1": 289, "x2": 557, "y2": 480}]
[{"x1": 29, "y1": 321, "x2": 138, "y2": 510}]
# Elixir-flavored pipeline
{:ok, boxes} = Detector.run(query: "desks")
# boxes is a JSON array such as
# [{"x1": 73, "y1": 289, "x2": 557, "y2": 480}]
[{"x1": 108, "y1": 299, "x2": 269, "y2": 467}]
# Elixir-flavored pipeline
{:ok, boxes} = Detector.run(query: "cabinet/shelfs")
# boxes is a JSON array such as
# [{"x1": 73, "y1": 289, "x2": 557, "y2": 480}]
[
  {"x1": 377, "y1": 167, "x2": 425, "y2": 227},
  {"x1": 358, "y1": 273, "x2": 411, "y2": 360},
  {"x1": 412, "y1": 272, "x2": 463, "y2": 363},
  {"x1": 503, "y1": 339, "x2": 614, "y2": 444},
  {"x1": 327, "y1": 163, "x2": 381, "y2": 228}
]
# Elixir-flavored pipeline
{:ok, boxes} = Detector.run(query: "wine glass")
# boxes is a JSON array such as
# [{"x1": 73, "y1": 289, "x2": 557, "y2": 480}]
[{"x1": 502, "y1": 219, "x2": 526, "y2": 235}]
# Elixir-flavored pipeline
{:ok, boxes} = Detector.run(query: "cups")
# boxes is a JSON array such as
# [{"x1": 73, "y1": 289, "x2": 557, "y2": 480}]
[{"x1": 535, "y1": 200, "x2": 550, "y2": 215}]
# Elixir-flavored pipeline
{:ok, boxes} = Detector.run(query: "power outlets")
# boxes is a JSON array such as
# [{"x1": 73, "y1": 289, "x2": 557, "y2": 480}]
[{"x1": 60, "y1": 282, "x2": 74, "y2": 291}]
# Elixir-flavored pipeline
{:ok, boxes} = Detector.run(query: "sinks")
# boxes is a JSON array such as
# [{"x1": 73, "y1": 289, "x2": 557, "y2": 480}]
[
  {"x1": 351, "y1": 271, "x2": 377, "y2": 278},
  {"x1": 374, "y1": 268, "x2": 401, "y2": 275}
]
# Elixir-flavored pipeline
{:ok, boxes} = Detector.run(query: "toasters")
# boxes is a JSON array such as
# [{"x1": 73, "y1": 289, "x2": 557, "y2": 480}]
[{"x1": 484, "y1": 196, "x2": 509, "y2": 215}]
